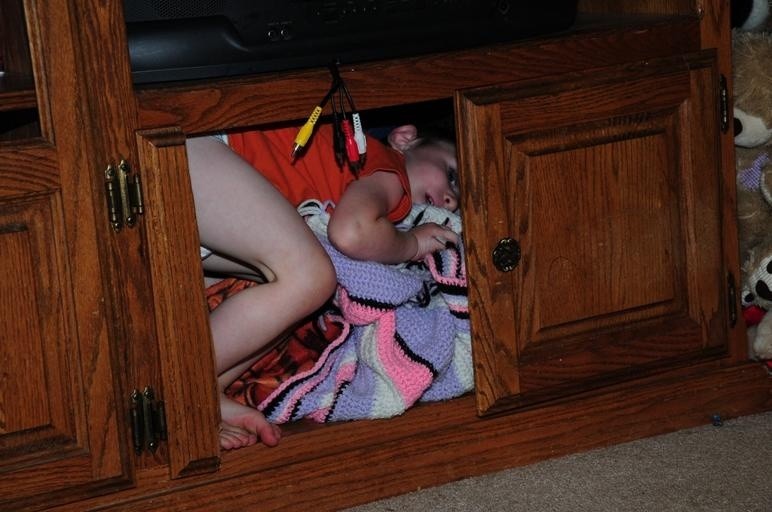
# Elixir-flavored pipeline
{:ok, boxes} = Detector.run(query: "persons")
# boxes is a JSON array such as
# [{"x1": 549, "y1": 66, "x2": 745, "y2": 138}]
[{"x1": 186, "y1": 118, "x2": 462, "y2": 452}]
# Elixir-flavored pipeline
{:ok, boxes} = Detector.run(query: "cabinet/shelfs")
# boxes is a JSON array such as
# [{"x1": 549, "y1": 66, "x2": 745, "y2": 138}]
[{"x1": 2, "y1": 0, "x2": 751, "y2": 512}]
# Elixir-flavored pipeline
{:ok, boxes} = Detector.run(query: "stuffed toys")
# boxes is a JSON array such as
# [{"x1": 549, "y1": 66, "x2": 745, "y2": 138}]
[{"x1": 729, "y1": 28, "x2": 771, "y2": 364}]
